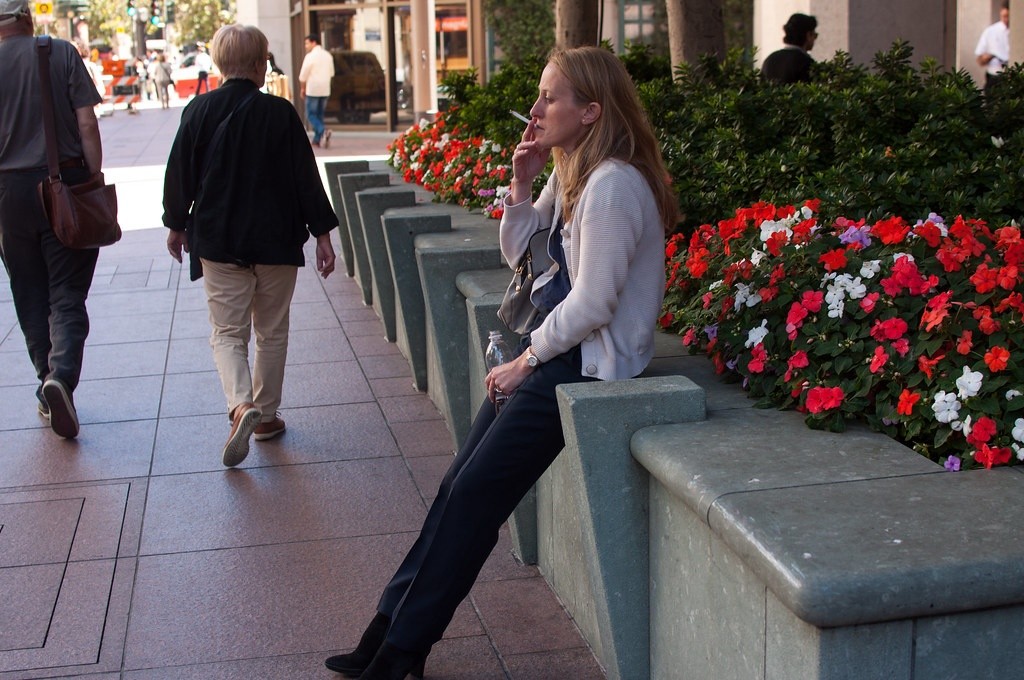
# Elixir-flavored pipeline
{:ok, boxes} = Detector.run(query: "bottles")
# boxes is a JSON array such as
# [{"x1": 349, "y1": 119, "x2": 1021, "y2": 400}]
[{"x1": 486, "y1": 330, "x2": 513, "y2": 415}]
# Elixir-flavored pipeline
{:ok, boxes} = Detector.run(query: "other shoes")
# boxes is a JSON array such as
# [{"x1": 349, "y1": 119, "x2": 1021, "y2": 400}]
[
  {"x1": 38, "y1": 401, "x2": 51, "y2": 419},
  {"x1": 43, "y1": 379, "x2": 80, "y2": 439},
  {"x1": 322, "y1": 129, "x2": 332, "y2": 150},
  {"x1": 311, "y1": 141, "x2": 320, "y2": 147}
]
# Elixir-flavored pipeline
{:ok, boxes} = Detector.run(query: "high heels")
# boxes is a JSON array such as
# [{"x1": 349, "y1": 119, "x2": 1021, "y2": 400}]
[
  {"x1": 326, "y1": 611, "x2": 393, "y2": 674},
  {"x1": 359, "y1": 635, "x2": 431, "y2": 680}
]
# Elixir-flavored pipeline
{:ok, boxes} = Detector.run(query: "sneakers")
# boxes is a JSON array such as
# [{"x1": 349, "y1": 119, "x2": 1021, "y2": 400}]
[
  {"x1": 253, "y1": 410, "x2": 285, "y2": 441},
  {"x1": 223, "y1": 402, "x2": 261, "y2": 467}
]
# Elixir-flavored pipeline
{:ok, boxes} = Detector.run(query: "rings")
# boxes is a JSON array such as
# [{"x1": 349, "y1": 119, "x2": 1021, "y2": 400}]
[
  {"x1": 495, "y1": 387, "x2": 503, "y2": 393},
  {"x1": 515, "y1": 143, "x2": 521, "y2": 150}
]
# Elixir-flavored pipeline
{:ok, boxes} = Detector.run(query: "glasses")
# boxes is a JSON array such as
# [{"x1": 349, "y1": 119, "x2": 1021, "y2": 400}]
[{"x1": 812, "y1": 32, "x2": 818, "y2": 40}]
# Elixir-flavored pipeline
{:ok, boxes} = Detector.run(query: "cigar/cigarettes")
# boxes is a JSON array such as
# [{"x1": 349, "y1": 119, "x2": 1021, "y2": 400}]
[{"x1": 509, "y1": 109, "x2": 537, "y2": 129}]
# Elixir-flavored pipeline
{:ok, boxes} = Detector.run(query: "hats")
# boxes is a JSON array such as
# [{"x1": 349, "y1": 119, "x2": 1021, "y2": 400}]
[{"x1": 0, "y1": 0, "x2": 30, "y2": 27}]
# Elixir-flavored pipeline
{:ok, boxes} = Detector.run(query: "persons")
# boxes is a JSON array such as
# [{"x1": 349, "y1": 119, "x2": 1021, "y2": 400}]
[
  {"x1": 975, "y1": 0, "x2": 1009, "y2": 104},
  {"x1": 161, "y1": 23, "x2": 337, "y2": 466},
  {"x1": 193, "y1": 47, "x2": 213, "y2": 96},
  {"x1": 299, "y1": 33, "x2": 335, "y2": 149},
  {"x1": 322, "y1": 46, "x2": 677, "y2": 680},
  {"x1": 0, "y1": 0, "x2": 123, "y2": 442},
  {"x1": 758, "y1": 13, "x2": 818, "y2": 82},
  {"x1": 71, "y1": 42, "x2": 173, "y2": 119}
]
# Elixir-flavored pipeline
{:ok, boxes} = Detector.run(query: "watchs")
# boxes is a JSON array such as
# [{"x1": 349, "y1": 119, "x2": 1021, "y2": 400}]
[{"x1": 526, "y1": 346, "x2": 538, "y2": 368}]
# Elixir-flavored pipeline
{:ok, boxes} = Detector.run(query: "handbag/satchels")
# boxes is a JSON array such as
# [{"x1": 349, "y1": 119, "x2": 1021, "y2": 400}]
[
  {"x1": 497, "y1": 226, "x2": 551, "y2": 335},
  {"x1": 39, "y1": 176, "x2": 121, "y2": 249},
  {"x1": 183, "y1": 213, "x2": 193, "y2": 252}
]
